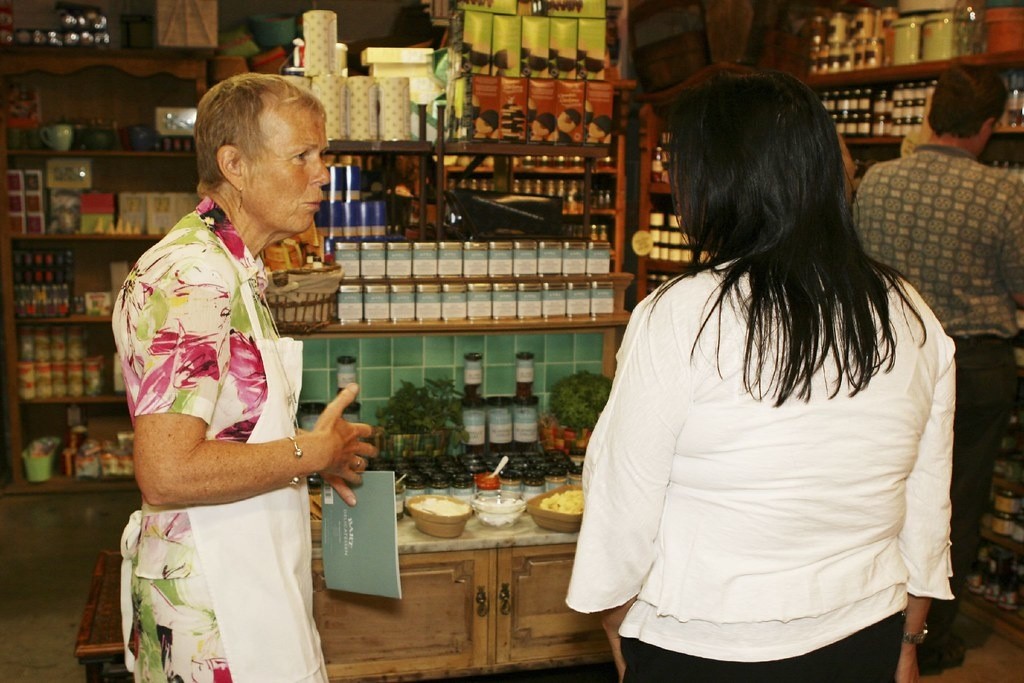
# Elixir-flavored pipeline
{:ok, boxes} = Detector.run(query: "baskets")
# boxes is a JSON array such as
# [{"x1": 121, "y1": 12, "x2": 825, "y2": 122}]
[{"x1": 266, "y1": 264, "x2": 344, "y2": 321}]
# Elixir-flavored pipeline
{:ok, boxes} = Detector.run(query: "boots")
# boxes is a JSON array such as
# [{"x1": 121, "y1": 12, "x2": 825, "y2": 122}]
[{"x1": 916, "y1": 621, "x2": 966, "y2": 675}]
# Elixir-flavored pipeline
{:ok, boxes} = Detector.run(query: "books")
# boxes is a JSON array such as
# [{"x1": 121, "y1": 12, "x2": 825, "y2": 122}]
[{"x1": 322, "y1": 472, "x2": 402, "y2": 599}]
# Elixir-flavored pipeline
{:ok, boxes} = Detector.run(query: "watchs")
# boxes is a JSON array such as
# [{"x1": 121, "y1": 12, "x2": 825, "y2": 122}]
[{"x1": 902, "y1": 621, "x2": 930, "y2": 644}]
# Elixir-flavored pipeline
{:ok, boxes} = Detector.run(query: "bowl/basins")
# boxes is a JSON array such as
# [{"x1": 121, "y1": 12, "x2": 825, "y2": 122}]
[
  {"x1": 406, "y1": 494, "x2": 473, "y2": 537},
  {"x1": 469, "y1": 490, "x2": 527, "y2": 530}
]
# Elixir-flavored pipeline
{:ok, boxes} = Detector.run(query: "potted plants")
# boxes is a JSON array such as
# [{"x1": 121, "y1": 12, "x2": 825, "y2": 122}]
[
  {"x1": 547, "y1": 371, "x2": 615, "y2": 454},
  {"x1": 370, "y1": 371, "x2": 471, "y2": 462}
]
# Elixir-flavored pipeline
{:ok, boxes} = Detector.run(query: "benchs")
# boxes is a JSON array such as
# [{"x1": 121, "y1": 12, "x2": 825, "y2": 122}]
[{"x1": 74, "y1": 539, "x2": 135, "y2": 682}]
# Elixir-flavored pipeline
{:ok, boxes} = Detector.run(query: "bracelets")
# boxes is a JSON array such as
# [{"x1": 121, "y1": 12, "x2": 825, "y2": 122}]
[{"x1": 286, "y1": 434, "x2": 303, "y2": 486}]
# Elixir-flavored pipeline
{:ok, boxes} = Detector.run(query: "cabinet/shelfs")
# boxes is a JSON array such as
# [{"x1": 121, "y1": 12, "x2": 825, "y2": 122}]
[{"x1": 1, "y1": 39, "x2": 1024, "y2": 493}]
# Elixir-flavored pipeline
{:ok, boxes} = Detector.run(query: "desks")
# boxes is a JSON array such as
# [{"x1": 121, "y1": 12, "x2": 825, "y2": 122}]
[{"x1": 308, "y1": 495, "x2": 630, "y2": 683}]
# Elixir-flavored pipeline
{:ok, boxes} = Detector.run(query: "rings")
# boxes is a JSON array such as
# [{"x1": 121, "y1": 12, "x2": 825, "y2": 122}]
[{"x1": 354, "y1": 459, "x2": 362, "y2": 470}]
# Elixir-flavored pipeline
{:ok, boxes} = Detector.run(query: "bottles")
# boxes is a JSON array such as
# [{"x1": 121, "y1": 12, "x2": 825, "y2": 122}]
[{"x1": 11, "y1": 250, "x2": 74, "y2": 317}]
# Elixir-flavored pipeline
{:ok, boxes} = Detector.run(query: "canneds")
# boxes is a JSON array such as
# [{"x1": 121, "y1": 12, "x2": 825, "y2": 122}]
[
  {"x1": 363, "y1": 447, "x2": 585, "y2": 518},
  {"x1": 16, "y1": 324, "x2": 103, "y2": 401},
  {"x1": 806, "y1": 4, "x2": 939, "y2": 137},
  {"x1": 977, "y1": 487, "x2": 1024, "y2": 541}
]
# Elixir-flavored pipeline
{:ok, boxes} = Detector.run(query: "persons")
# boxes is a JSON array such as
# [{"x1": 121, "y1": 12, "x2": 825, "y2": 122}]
[
  {"x1": 111, "y1": 70, "x2": 379, "y2": 683},
  {"x1": 853, "y1": 63, "x2": 1024, "y2": 672},
  {"x1": 563, "y1": 68, "x2": 956, "y2": 683}
]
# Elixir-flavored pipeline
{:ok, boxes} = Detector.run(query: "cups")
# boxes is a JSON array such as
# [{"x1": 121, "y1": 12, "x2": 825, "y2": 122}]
[{"x1": 40, "y1": 122, "x2": 154, "y2": 149}]
